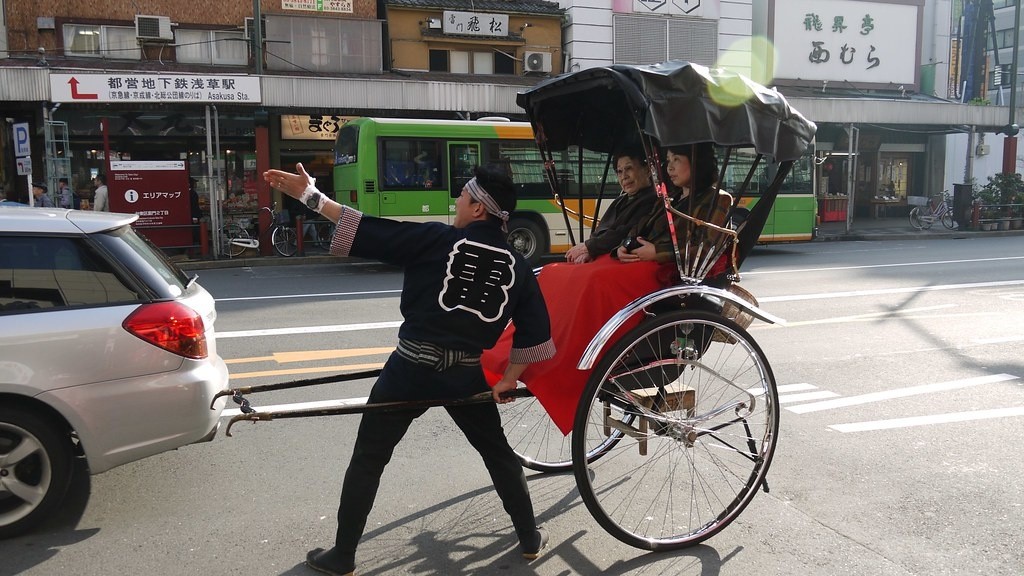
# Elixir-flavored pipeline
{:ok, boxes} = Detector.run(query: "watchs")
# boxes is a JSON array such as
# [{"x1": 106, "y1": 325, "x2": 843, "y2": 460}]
[{"x1": 306, "y1": 192, "x2": 323, "y2": 211}]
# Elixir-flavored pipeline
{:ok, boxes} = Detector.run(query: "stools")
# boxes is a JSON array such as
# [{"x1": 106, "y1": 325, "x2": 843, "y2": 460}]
[{"x1": 602, "y1": 382, "x2": 695, "y2": 455}]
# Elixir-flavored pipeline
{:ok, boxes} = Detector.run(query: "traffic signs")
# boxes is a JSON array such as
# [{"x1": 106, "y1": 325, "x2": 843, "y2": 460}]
[{"x1": 17, "y1": 158, "x2": 33, "y2": 175}]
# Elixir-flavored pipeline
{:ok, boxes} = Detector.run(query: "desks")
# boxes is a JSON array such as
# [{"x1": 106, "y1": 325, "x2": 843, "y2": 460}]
[
  {"x1": 871, "y1": 200, "x2": 901, "y2": 220},
  {"x1": 818, "y1": 196, "x2": 848, "y2": 222}
]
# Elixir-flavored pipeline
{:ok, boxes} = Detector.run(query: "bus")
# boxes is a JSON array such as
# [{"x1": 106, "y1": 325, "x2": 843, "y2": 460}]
[{"x1": 331, "y1": 117, "x2": 834, "y2": 266}]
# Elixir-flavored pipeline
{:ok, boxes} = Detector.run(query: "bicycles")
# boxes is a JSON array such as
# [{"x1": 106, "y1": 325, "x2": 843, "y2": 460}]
[
  {"x1": 909, "y1": 189, "x2": 959, "y2": 231},
  {"x1": 217, "y1": 202, "x2": 298, "y2": 257}
]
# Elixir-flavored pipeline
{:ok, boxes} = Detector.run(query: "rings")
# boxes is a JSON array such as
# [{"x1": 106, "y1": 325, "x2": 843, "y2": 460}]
[{"x1": 278, "y1": 182, "x2": 281, "y2": 188}]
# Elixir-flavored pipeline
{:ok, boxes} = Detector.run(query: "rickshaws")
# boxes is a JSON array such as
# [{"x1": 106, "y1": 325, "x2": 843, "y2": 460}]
[{"x1": 211, "y1": 58, "x2": 819, "y2": 552}]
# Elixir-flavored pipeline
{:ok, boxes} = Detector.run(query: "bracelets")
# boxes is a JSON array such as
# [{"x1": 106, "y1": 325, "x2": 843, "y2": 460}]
[{"x1": 299, "y1": 186, "x2": 329, "y2": 214}]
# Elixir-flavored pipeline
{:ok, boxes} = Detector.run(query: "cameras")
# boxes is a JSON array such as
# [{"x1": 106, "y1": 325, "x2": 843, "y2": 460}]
[{"x1": 625, "y1": 236, "x2": 641, "y2": 254}]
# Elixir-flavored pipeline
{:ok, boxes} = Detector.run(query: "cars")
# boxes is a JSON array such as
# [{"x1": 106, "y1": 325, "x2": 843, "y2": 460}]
[{"x1": 0, "y1": 204, "x2": 230, "y2": 541}]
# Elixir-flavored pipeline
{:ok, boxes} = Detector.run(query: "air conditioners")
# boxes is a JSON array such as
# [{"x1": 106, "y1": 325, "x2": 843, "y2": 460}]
[
  {"x1": 134, "y1": 14, "x2": 178, "y2": 40},
  {"x1": 522, "y1": 51, "x2": 553, "y2": 76},
  {"x1": 244, "y1": 16, "x2": 267, "y2": 40}
]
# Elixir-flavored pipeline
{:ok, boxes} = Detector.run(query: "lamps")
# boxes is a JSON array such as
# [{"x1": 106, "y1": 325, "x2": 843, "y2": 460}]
[
  {"x1": 419, "y1": 18, "x2": 434, "y2": 25},
  {"x1": 520, "y1": 22, "x2": 532, "y2": 32}
]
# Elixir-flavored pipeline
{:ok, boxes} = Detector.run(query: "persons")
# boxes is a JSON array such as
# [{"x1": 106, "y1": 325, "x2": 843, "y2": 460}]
[
  {"x1": 93, "y1": 175, "x2": 109, "y2": 211},
  {"x1": 58, "y1": 178, "x2": 74, "y2": 208},
  {"x1": 890, "y1": 181, "x2": 895, "y2": 196},
  {"x1": 565, "y1": 144, "x2": 730, "y2": 266},
  {"x1": 189, "y1": 178, "x2": 201, "y2": 242},
  {"x1": 262, "y1": 162, "x2": 556, "y2": 576},
  {"x1": 31, "y1": 182, "x2": 55, "y2": 208}
]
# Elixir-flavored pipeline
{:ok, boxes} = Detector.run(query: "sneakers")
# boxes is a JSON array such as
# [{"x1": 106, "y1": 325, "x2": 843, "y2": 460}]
[
  {"x1": 522, "y1": 526, "x2": 550, "y2": 560},
  {"x1": 306, "y1": 547, "x2": 356, "y2": 576}
]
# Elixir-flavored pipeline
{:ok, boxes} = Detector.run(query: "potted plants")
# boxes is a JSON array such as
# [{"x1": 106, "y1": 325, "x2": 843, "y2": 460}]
[{"x1": 969, "y1": 172, "x2": 1024, "y2": 230}]
